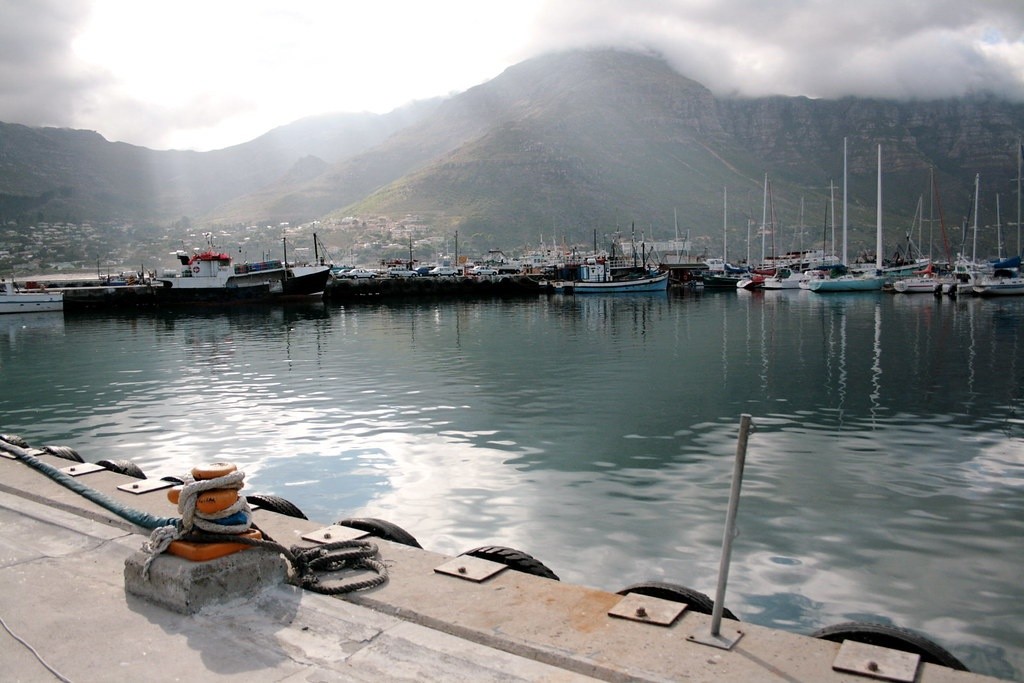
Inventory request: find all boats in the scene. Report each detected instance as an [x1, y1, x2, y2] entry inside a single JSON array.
[[52, 137, 1024, 315], [0, 273, 63, 314]]
